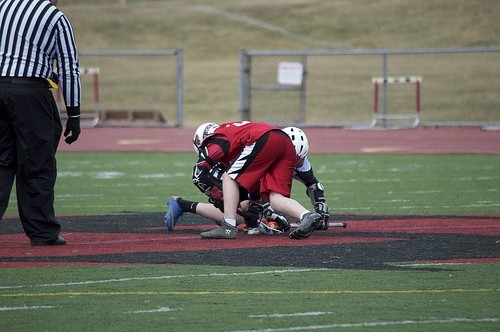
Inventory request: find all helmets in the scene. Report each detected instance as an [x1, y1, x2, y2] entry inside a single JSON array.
[[282, 126, 310, 160], [193, 122, 220, 160]]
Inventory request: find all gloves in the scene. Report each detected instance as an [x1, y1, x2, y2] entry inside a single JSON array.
[[213, 191, 224, 214], [244, 206, 262, 228], [265, 213, 289, 231], [312, 202, 330, 231], [64, 116, 82, 145]]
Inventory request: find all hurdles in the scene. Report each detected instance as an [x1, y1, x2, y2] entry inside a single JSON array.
[[55, 66, 100, 128], [342, 75, 423, 131]]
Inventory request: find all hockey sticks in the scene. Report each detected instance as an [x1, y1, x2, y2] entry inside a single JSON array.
[[236, 221, 347, 231]]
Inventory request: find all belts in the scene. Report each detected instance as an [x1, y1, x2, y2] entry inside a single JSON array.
[[0, 76, 48, 84]]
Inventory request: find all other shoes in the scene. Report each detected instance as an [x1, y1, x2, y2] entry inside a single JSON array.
[[30, 235, 68, 246]]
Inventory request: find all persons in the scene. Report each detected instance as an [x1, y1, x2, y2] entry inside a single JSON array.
[[164, 120, 330, 239], [0, 0, 82, 247]]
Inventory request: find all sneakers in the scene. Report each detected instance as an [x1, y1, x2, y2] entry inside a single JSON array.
[[288, 213, 323, 240], [164, 195, 184, 232], [200, 218, 239, 239]]
